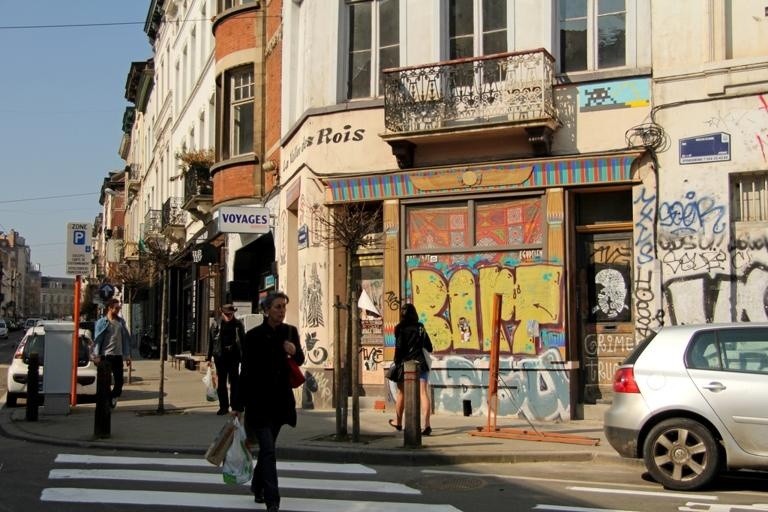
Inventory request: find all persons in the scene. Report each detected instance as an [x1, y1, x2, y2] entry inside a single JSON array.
[[205, 303, 246, 416], [92, 297, 132, 409], [230, 290, 305, 510], [386, 300, 433, 437]]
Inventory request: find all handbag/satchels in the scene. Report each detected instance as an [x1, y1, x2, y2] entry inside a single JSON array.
[[88, 339, 105, 367], [285, 324, 306, 388], [205, 418, 237, 467], [385, 366, 399, 381], [222, 417, 254, 485]]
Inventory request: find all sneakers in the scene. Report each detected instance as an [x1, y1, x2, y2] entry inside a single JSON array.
[[110, 396, 118, 409]]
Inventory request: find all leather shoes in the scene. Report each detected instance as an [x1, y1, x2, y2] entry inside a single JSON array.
[[389, 420, 402, 431], [216, 408, 229, 415], [254, 488, 266, 503], [267, 502, 280, 512], [421, 426, 432, 435]]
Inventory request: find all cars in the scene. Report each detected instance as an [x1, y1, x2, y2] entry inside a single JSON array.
[[604, 322, 767, 491], [0, 315, 98, 408]]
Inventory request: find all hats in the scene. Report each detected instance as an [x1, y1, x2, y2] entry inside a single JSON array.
[[218, 304, 238, 314]]
